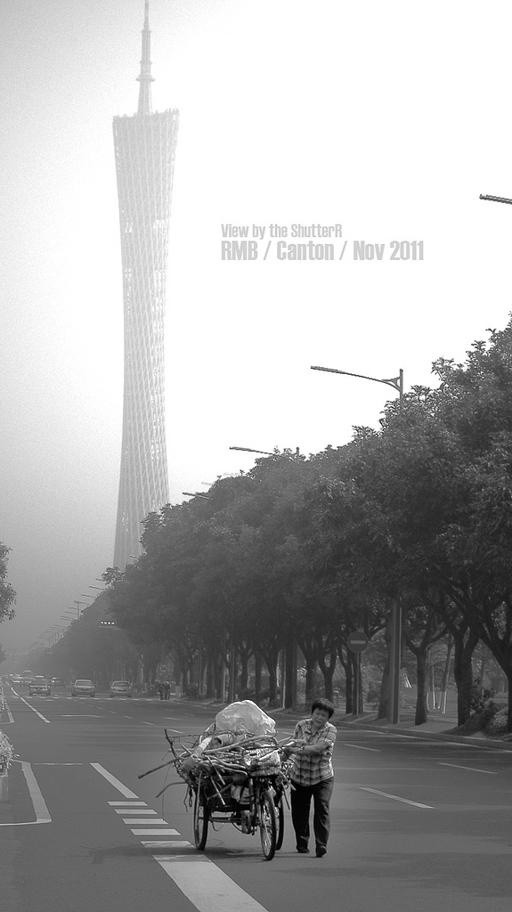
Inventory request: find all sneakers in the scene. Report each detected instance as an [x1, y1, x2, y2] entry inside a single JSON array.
[[296, 845, 328, 857]]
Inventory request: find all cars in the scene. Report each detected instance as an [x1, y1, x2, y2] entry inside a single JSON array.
[[71, 680, 96, 697], [110, 680, 132, 698], [10, 670, 62, 696]]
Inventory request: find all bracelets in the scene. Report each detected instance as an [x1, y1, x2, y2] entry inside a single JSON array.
[[299, 746, 304, 755]]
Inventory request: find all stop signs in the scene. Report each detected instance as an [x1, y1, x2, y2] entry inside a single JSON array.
[[347, 632, 369, 652]]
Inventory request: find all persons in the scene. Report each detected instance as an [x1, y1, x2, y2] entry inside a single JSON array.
[[157, 679, 172, 700], [280, 699, 338, 861]]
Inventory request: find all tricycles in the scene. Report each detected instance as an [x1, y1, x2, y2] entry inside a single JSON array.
[[192, 744, 296, 860]]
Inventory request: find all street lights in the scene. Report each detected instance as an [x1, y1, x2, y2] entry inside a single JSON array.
[[311, 364, 404, 728], [229, 445, 363, 715]]
